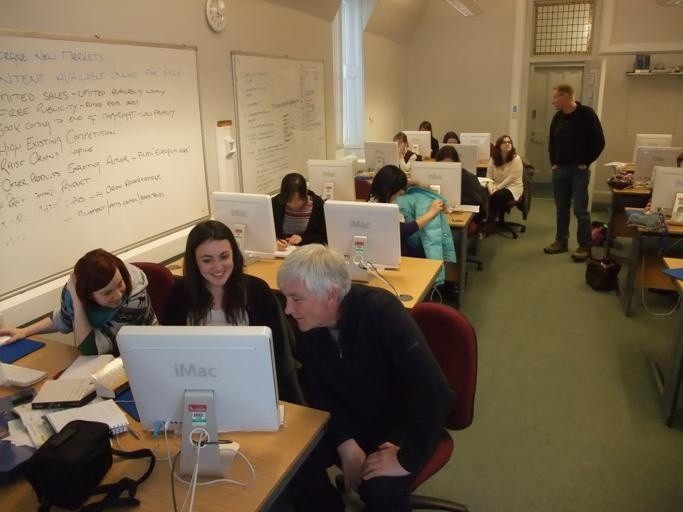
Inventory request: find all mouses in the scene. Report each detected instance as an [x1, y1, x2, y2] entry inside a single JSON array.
[[0, 335, 12, 347]]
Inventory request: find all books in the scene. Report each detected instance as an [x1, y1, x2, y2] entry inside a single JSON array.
[[659, 267, 683, 281], [0, 352, 139, 451]]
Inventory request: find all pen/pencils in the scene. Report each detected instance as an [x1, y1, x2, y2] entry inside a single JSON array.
[[52, 356, 74, 379], [126, 424, 142, 441]]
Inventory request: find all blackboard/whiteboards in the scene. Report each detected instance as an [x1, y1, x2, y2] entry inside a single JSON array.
[[0, 29, 212, 303], [232, 50, 327, 196]]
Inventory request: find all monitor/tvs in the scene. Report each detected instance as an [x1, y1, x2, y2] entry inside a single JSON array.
[[323, 200, 400, 282], [402, 130, 431, 159], [411, 161, 462, 213], [363, 141, 399, 171], [116, 326, 280, 477], [634, 146, 683, 178], [650, 167, 683, 225], [460, 133, 490, 162], [439, 143, 477, 176], [212, 191, 275, 265], [307, 160, 356, 202], [634, 134, 672, 163]]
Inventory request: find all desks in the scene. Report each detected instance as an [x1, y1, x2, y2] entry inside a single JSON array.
[[602, 160, 683, 427]]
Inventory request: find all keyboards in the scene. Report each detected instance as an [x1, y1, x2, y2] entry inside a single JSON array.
[[245, 243, 297, 259], [1, 363, 48, 389], [368, 264, 400, 270]]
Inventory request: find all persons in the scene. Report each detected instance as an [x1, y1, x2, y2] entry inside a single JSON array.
[[643, 152, 682, 213], [393, 121, 523, 260], [362, 166, 443, 256], [543, 82, 605, 260], [277, 239, 453, 512], [1, 248, 161, 359], [166, 221, 301, 405], [269, 172, 326, 250]]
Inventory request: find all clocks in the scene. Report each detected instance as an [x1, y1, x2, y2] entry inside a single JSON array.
[[204, 0, 228, 33]]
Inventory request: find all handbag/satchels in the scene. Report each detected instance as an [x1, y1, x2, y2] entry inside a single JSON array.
[[585, 262, 618, 291], [24, 420, 155, 512]]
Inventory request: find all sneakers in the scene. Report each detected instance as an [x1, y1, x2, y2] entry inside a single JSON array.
[[544, 241, 568, 253], [571, 247, 591, 259]]
